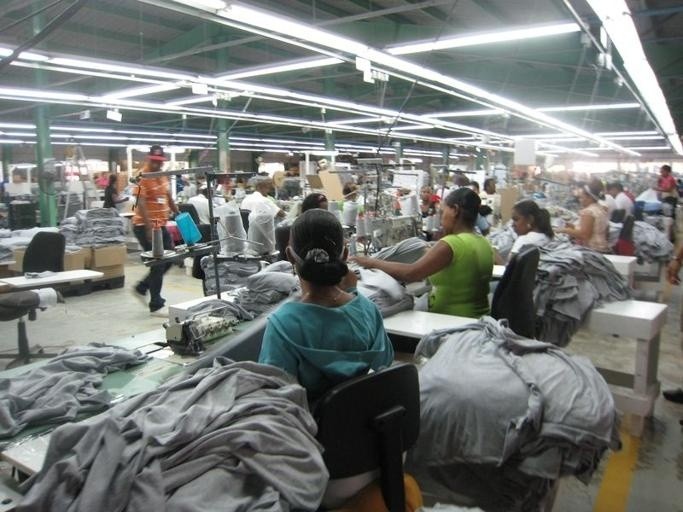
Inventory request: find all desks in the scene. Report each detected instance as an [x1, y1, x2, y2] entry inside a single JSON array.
[[0, 215, 683, 512]]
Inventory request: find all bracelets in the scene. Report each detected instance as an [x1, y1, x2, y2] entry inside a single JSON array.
[[671, 256, 680, 260]]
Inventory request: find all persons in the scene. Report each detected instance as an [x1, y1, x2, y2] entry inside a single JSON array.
[[131, 145, 183, 310], [397, 174, 502, 237], [512, 172, 552, 200], [664, 225, 682, 285], [175, 172, 218, 225], [347, 188, 495, 317], [103, 174, 130, 209], [654, 165, 679, 241], [557, 181, 636, 254], [301, 193, 328, 213], [242, 176, 289, 253], [256, 206, 407, 511], [343, 182, 364, 226], [506, 202, 554, 263]]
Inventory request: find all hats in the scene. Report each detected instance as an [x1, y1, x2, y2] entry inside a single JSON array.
[[145, 145, 170, 161]]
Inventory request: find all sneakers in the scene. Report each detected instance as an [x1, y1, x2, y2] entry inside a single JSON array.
[[132, 285, 149, 307], [149, 305, 169, 317]]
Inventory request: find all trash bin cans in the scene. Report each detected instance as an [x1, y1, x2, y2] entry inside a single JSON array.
[[7, 200, 36, 230], [174, 212, 201, 244]]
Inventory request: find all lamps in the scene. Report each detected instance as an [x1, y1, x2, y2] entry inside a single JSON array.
[[0, 0, 683, 158]]
